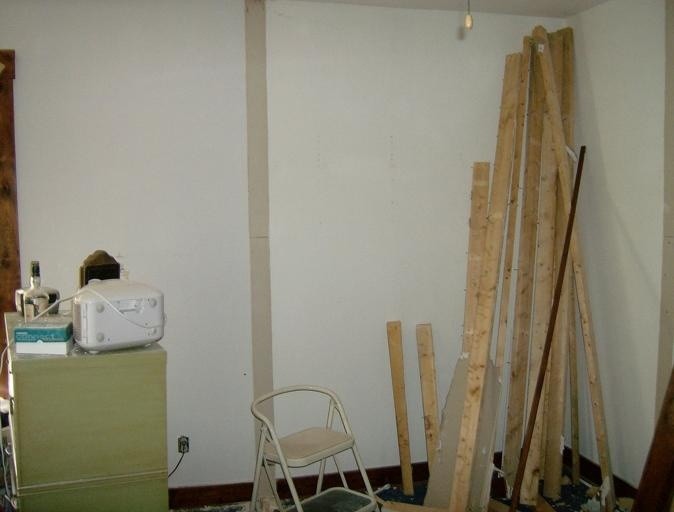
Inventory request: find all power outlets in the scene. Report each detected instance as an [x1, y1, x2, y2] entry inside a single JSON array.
[[177, 436, 189, 452]]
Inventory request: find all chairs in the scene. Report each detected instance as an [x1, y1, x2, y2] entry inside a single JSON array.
[[249, 384, 380, 512]]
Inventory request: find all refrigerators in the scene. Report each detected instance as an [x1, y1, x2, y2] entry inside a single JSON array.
[[3, 309, 168, 511]]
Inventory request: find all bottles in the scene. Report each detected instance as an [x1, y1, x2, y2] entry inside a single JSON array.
[[23, 261, 49, 323]]
